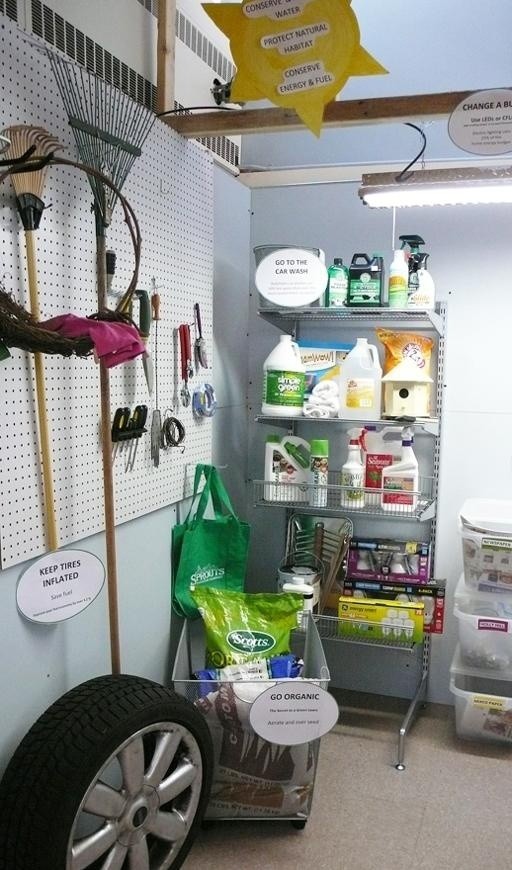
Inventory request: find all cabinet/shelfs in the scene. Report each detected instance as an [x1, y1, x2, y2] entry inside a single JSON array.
[[250, 298, 448, 771]]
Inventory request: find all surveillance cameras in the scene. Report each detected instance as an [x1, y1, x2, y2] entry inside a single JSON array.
[[211, 75, 245, 105]]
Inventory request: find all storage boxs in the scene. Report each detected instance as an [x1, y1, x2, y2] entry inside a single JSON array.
[[454, 573, 512, 671], [460, 499, 512, 593], [449, 643, 512, 747]]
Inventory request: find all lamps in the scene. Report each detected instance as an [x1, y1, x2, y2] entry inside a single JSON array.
[[357, 167, 511, 208]]
